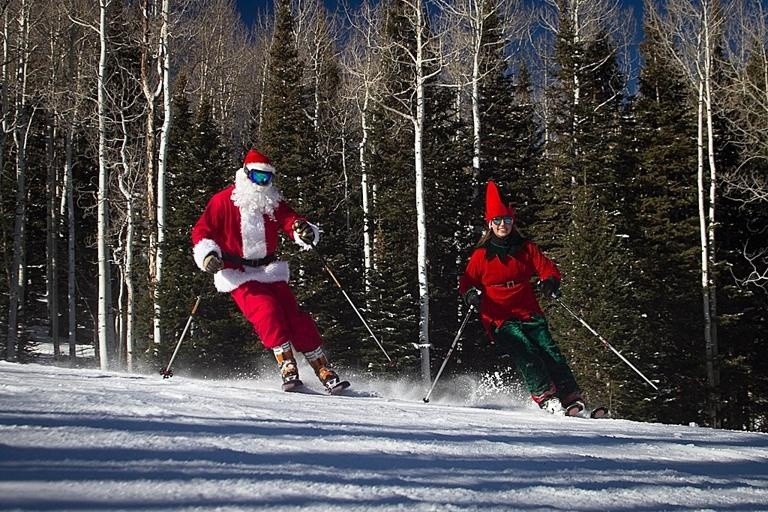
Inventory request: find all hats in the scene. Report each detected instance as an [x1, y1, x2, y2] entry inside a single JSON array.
[[242, 147, 277, 172], [484, 179, 516, 224]]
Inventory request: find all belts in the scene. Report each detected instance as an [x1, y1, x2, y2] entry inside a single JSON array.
[[493, 279, 522, 288]]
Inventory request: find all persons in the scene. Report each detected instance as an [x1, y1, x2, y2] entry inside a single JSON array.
[[193, 148, 341, 390], [459, 179, 589, 416]]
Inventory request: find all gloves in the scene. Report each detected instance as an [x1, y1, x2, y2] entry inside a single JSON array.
[[539, 276, 561, 297], [204, 256, 224, 274], [291, 220, 315, 243], [465, 288, 480, 311]]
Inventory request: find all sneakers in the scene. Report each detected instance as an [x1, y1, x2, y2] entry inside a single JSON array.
[[538, 395, 564, 414], [571, 398, 587, 414]]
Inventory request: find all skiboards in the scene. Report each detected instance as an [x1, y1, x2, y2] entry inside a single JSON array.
[[281, 379, 351, 395], [565, 403, 608, 420]]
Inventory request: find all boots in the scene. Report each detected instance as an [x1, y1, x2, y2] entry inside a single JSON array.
[[303, 347, 339, 386], [272, 342, 300, 381]]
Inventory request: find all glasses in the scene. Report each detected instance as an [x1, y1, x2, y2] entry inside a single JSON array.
[[249, 169, 274, 185], [493, 216, 514, 226]]
